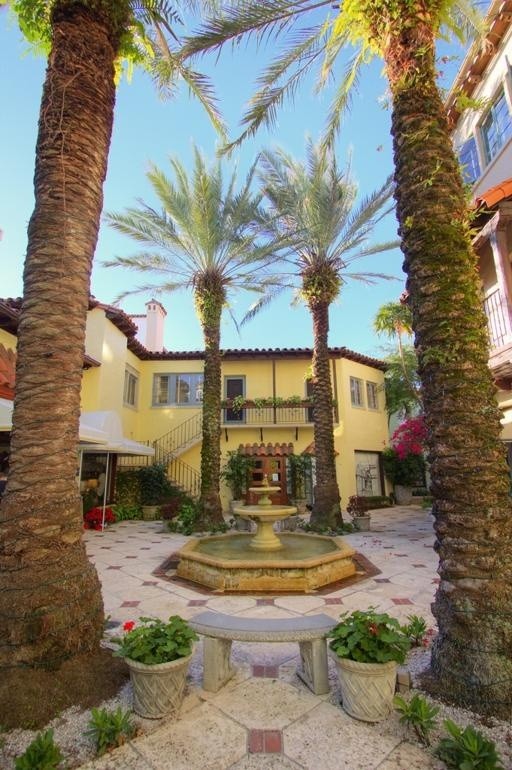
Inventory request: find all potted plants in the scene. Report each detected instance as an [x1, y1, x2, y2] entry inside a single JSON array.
[[279, 449, 315, 515], [134, 462, 170, 521], [381, 447, 427, 507], [219, 449, 257, 515]]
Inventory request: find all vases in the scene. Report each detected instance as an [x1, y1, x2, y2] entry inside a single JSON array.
[[333, 656, 400, 722], [162, 518, 181, 531], [351, 515, 373, 530], [124, 648, 193, 722]]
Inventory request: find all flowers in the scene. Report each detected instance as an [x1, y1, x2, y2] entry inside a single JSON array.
[[106, 615, 203, 666], [347, 495, 371, 515], [157, 498, 182, 518], [325, 601, 440, 664]]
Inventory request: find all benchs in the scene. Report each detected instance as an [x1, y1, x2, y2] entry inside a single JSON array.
[[186, 610, 340, 697]]
